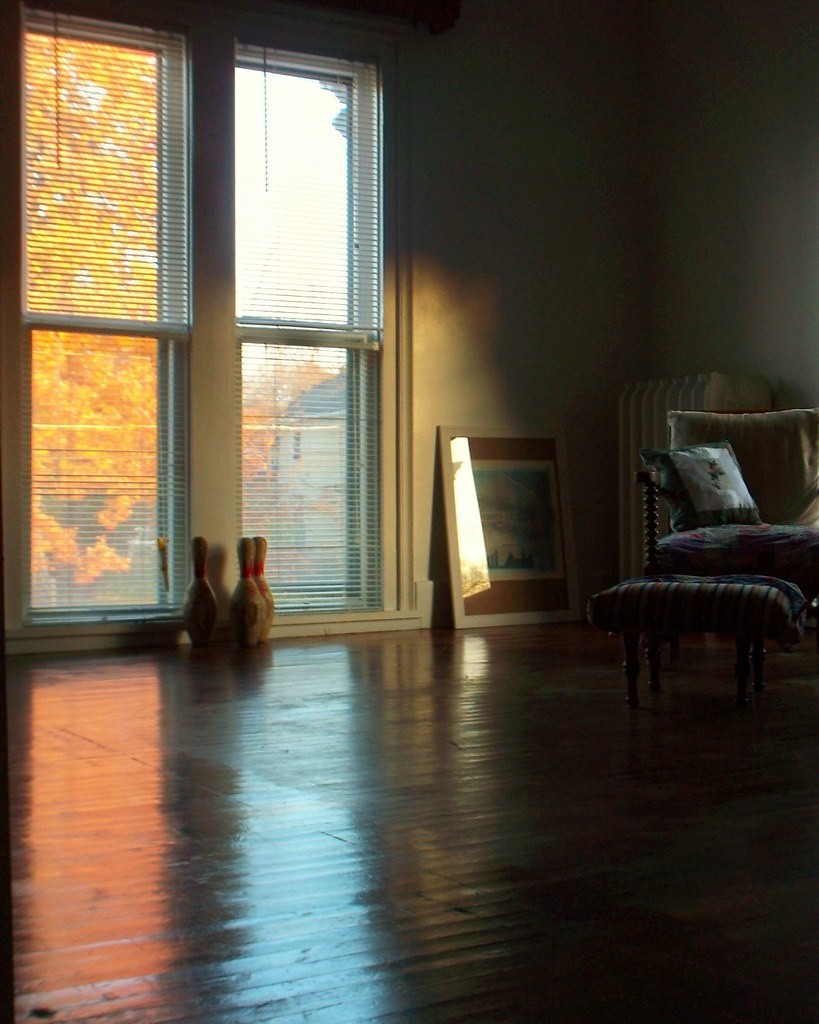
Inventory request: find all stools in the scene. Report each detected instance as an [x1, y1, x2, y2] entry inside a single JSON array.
[[587, 573, 807, 707]]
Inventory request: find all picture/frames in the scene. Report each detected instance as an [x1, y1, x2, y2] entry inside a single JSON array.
[[436, 425, 582, 629]]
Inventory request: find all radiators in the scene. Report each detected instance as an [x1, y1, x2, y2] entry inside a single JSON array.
[[618, 371, 739, 587]]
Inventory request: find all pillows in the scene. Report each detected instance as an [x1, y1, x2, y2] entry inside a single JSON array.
[[639, 438, 764, 535]]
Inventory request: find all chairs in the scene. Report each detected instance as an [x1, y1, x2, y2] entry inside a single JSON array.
[[635, 407, 819, 695]]
